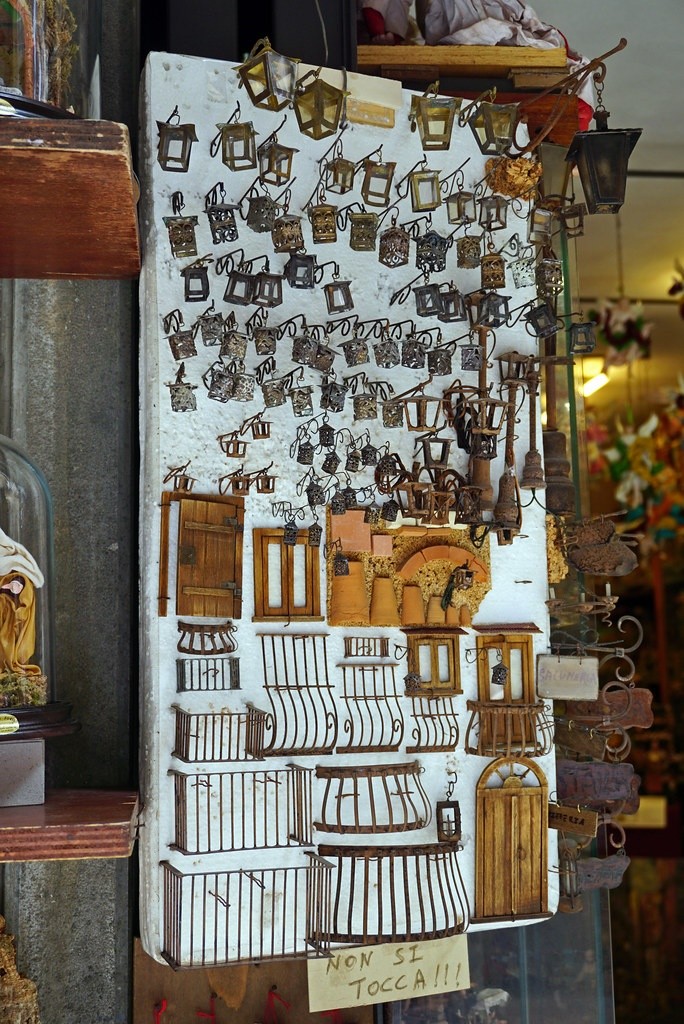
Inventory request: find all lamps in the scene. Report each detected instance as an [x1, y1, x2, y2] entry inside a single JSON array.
[[505, 38, 644, 216]]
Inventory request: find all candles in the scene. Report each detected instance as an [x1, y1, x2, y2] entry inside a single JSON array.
[[606, 581, 611, 598], [550, 588, 555, 600], [580, 592, 585, 602]]
